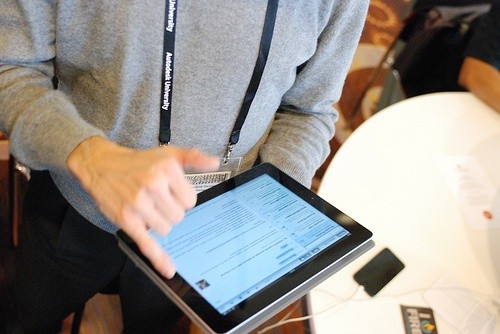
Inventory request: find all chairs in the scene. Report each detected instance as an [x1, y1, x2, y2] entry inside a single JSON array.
[[371, 71, 407, 110], [454, 58, 500, 112]]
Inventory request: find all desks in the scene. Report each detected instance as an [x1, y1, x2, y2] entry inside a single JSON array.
[[304, 91, 499, 334]]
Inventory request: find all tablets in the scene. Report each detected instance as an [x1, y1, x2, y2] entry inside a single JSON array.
[[116, 162, 373, 334]]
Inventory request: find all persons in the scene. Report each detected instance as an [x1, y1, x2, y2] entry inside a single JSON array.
[[0, 0, 370, 334]]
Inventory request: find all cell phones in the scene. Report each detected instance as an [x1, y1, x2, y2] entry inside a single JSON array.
[[354, 248, 405, 297]]
